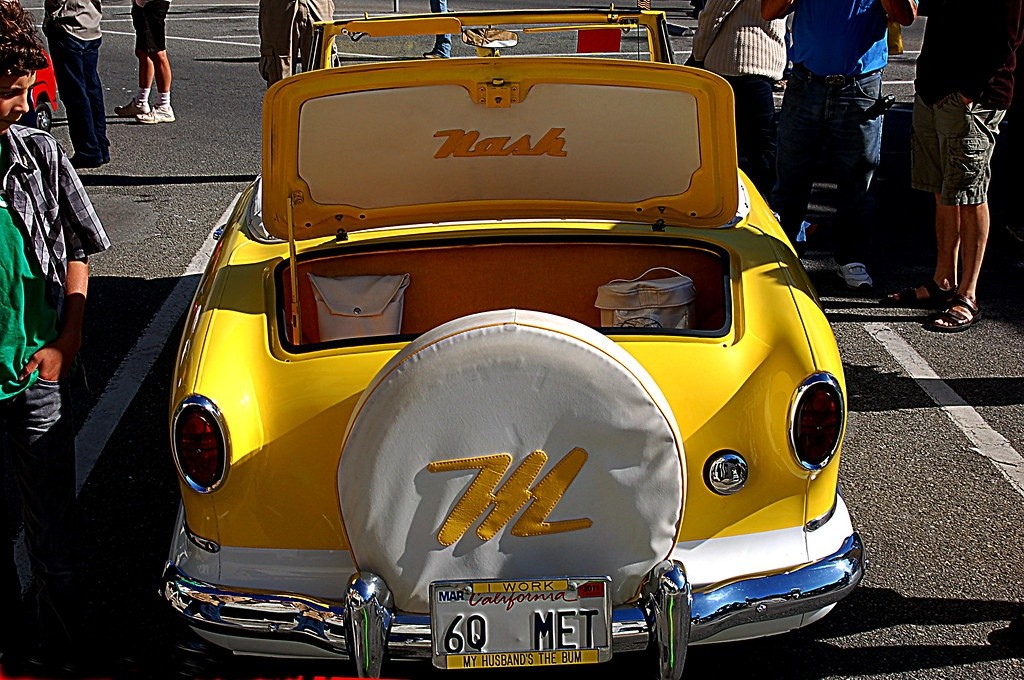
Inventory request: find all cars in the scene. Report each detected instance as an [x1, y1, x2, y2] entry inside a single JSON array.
[[156, 4, 865, 680], [18, 45, 60, 136]]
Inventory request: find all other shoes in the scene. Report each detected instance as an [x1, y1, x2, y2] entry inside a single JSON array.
[[69, 157, 103, 168], [104, 158, 110, 163]]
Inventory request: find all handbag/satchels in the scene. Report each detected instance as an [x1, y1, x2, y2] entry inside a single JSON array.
[[682, 51, 709, 69]]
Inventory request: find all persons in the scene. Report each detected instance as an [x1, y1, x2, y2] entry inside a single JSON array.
[[424, 0, 452, 59], [114, 0, 176, 123], [43, 0, 110, 169], [0, 0, 112, 664], [258, 0, 342, 89], [691, 0, 1024, 332]]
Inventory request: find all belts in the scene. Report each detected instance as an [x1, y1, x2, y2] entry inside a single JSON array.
[[797, 64, 878, 87]]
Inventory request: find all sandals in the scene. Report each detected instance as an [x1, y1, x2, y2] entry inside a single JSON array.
[[934, 294, 984, 332], [887, 279, 960, 306]]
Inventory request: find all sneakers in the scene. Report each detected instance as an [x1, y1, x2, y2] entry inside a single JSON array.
[[113, 98, 152, 117], [837, 263, 873, 292], [134, 103, 176, 124]]
